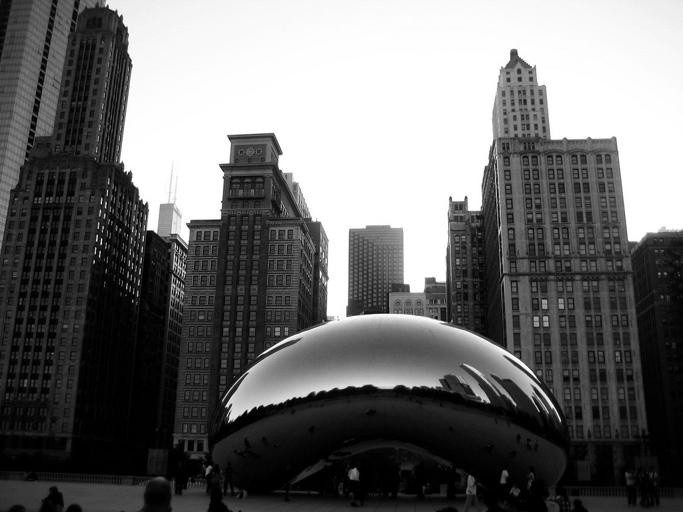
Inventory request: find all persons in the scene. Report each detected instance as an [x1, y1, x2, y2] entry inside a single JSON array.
[[623, 465, 662, 508], [347, 464, 364, 507], [7, 462, 236, 511], [462, 466, 588, 512]]
